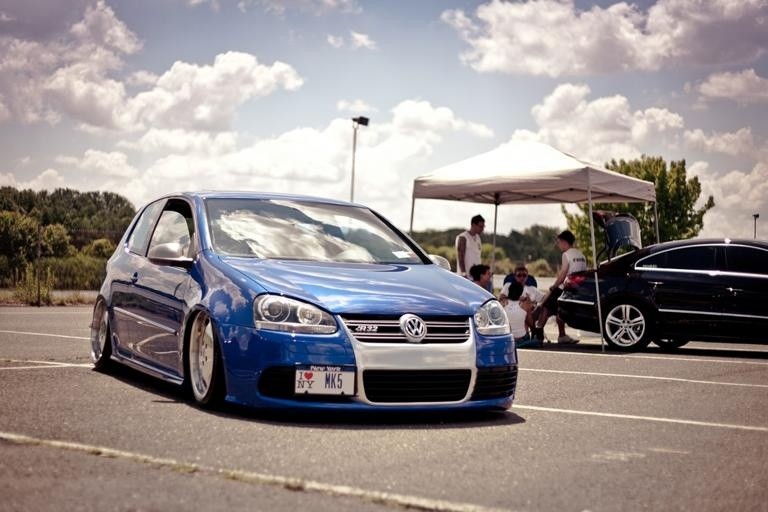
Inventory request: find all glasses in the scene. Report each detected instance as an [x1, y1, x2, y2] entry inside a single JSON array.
[[515, 273, 527, 278]]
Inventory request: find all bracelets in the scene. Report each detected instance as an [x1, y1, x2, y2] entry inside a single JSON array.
[[461, 272, 468, 276]]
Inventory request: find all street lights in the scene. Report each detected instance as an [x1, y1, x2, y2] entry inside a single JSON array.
[[752, 213, 760, 239], [350, 116, 369, 203]]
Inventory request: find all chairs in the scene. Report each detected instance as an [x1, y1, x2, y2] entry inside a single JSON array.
[[503, 273, 537, 289]]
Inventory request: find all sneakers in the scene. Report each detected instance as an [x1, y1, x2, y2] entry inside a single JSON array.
[[557, 334, 580, 344]]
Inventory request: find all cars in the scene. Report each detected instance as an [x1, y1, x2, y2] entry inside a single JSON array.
[[556, 235, 768, 354], [88, 189, 519, 417]]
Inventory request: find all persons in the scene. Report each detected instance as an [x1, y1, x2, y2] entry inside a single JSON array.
[[524, 230, 586, 347], [500, 282, 534, 347], [470, 264, 494, 294], [500, 267, 580, 344], [455, 214, 485, 282]]
